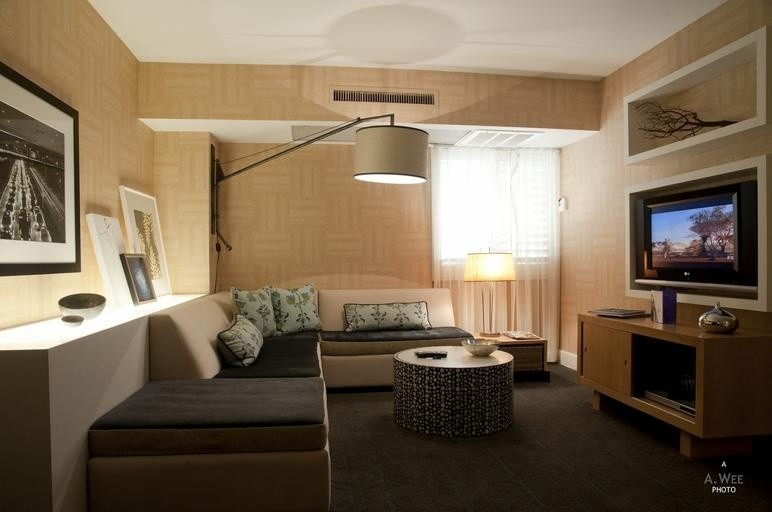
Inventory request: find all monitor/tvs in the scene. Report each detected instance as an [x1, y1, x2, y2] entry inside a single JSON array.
[[635, 179, 758, 288]]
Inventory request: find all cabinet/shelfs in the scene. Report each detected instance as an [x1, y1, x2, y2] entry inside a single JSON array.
[[622, 24, 767, 166], [623, 152, 770, 315], [573, 306, 772, 461], [479, 329, 551, 383]]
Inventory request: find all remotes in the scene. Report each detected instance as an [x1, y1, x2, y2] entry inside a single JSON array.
[[416, 352, 447, 358]]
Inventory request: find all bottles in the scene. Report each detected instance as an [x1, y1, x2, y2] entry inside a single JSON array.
[[697, 301, 739, 333]]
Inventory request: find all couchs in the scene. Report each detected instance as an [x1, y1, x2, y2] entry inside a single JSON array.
[[318, 285, 473, 391], [83, 287, 334, 509]]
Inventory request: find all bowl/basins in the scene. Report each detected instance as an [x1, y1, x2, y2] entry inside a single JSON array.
[[461, 338, 508, 356], [59, 294, 106, 319], [62, 315, 82, 327]]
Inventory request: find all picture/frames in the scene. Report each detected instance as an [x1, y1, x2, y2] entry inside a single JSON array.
[[119, 182, 172, 297], [0, 57, 82, 276], [119, 252, 157, 307]]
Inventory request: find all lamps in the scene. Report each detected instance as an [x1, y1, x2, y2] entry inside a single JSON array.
[[210, 112, 429, 250], [459, 247, 529, 338]]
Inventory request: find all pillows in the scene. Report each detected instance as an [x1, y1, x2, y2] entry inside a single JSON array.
[[217, 312, 265, 369], [268, 279, 321, 336], [231, 283, 281, 339], [343, 301, 433, 333]]
